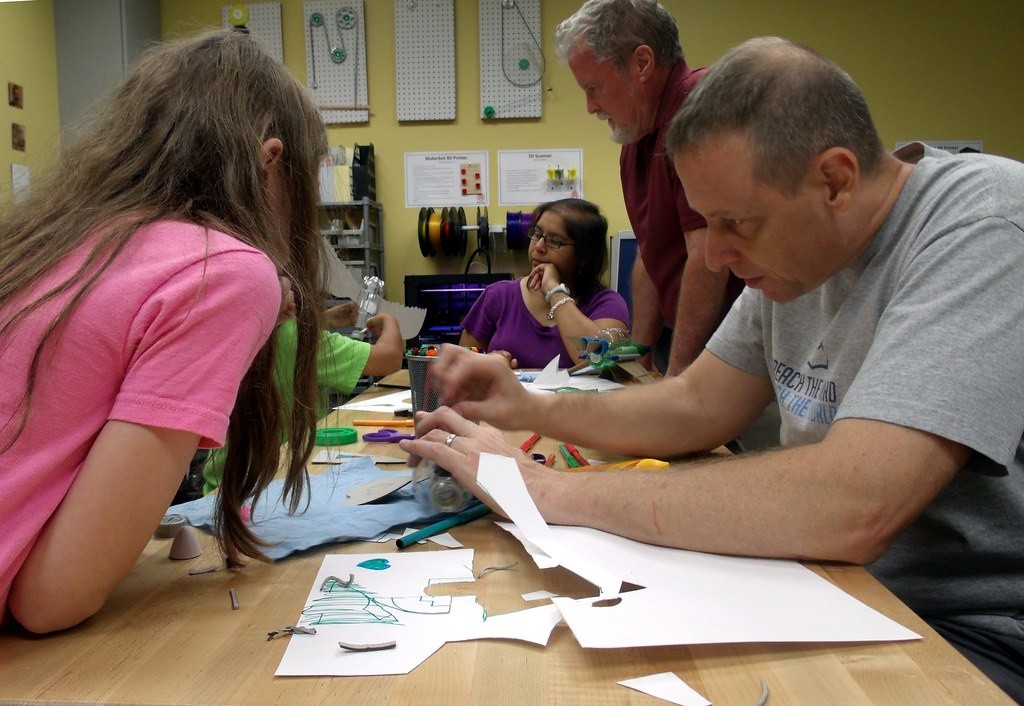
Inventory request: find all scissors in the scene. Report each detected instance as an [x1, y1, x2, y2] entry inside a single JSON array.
[[362, 428, 419, 445]]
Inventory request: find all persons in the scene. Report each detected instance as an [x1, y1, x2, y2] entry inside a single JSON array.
[[391, 31, 1023, 704], [0, 22, 347, 660], [195, 289, 417, 505], [538, 0, 761, 420], [451, 191, 634, 376]]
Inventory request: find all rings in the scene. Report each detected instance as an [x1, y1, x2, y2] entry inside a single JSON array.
[[444, 433, 457, 447]]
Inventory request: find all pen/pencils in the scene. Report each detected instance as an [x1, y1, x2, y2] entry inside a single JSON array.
[[564, 440, 592, 466], [518, 433, 546, 453], [395, 503, 497, 549], [404, 344, 479, 416], [352, 419, 416, 428], [558, 443, 580, 469]]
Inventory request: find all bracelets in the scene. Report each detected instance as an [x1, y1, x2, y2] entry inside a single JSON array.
[[547, 297, 576, 323]]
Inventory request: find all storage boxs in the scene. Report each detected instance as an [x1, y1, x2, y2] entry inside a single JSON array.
[[407, 344, 488, 432]]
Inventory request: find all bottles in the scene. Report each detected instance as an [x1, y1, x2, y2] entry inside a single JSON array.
[[349, 276, 385, 340], [412, 458, 473, 514]]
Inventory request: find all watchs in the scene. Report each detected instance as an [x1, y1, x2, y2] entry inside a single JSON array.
[[545, 283, 572, 305]]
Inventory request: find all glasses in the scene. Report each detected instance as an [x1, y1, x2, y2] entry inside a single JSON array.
[[527, 227, 575, 250]]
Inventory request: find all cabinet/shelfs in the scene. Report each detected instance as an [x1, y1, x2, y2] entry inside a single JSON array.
[[311, 198, 384, 411]]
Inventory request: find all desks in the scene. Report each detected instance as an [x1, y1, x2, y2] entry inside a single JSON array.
[[1, 368, 1019, 705]]
